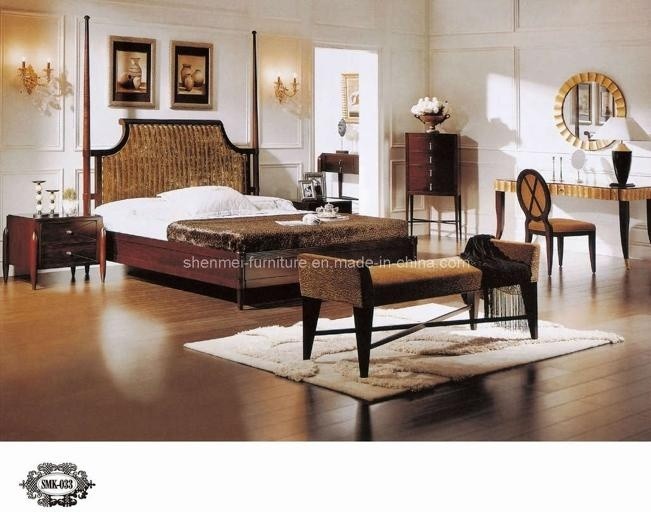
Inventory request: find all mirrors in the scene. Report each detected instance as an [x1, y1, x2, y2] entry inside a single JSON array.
[[554, 71, 627, 154]]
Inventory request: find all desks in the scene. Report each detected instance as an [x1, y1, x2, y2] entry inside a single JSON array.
[[318, 152, 360, 201], [494, 177, 651, 268], [403, 131, 461, 242]]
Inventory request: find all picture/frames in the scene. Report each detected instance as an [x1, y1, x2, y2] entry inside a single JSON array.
[[573, 82, 592, 124], [170, 40, 214, 110], [342, 72, 359, 125], [304, 172, 326, 198], [299, 180, 316, 200], [598, 84, 617, 125], [109, 35, 157, 109]]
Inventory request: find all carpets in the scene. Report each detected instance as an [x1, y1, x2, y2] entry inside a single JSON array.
[[184, 303, 623, 404]]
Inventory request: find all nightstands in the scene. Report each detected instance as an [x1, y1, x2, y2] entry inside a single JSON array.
[[291, 200, 354, 215], [3, 214, 107, 289]]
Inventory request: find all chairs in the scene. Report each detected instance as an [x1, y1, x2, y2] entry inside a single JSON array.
[[516, 169, 597, 276]]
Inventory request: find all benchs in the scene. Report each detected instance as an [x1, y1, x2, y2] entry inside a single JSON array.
[[300, 236, 540, 378]]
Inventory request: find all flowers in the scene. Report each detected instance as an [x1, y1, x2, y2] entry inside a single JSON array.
[[411, 95, 453, 114]]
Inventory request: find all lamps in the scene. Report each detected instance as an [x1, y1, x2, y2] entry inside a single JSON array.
[[274, 66, 287, 108], [17, 59, 37, 97], [589, 118, 651, 187], [286, 72, 298, 98], [42, 59, 52, 84]]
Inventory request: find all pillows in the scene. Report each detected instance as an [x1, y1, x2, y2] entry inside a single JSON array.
[[156, 185, 260, 217]]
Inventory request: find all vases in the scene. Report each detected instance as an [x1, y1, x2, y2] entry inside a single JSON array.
[[418, 115, 450, 131]]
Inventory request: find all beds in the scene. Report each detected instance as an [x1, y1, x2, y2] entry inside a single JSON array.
[[82, 16, 418, 311]]
